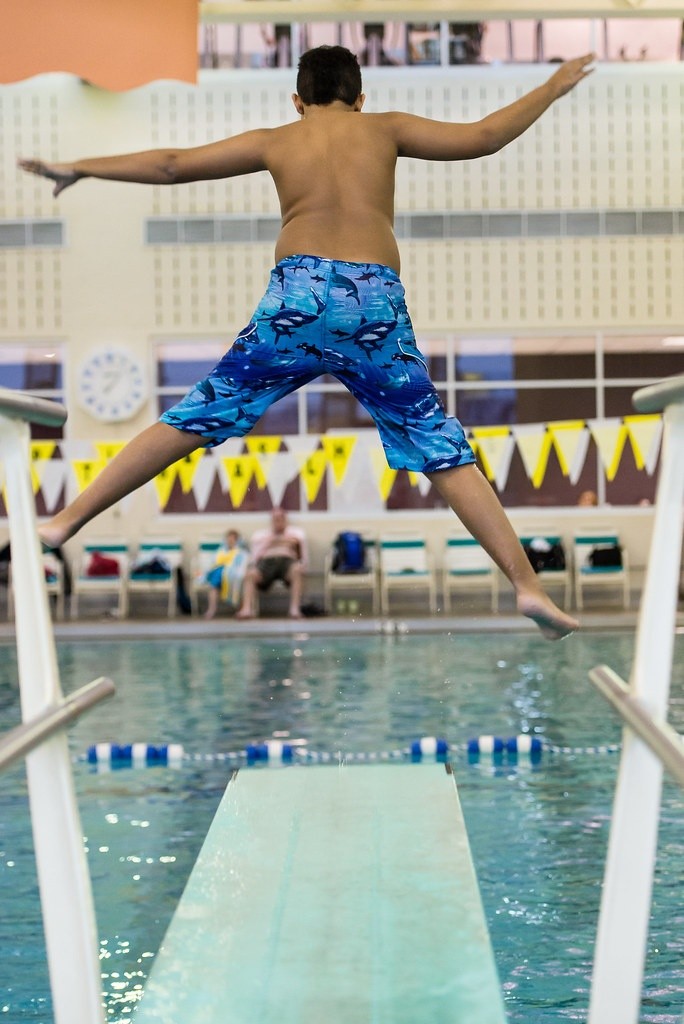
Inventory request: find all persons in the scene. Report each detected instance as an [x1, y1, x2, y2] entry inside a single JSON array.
[[17, 42, 599, 643], [199, 506, 308, 621]]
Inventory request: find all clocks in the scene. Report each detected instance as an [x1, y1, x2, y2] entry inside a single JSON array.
[[75, 347, 148, 421]]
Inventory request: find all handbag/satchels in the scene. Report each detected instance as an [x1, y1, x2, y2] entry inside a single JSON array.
[[589, 545, 623, 569], [528, 544, 565, 575]]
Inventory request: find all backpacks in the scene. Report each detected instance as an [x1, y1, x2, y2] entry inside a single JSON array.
[[332, 532, 368, 574]]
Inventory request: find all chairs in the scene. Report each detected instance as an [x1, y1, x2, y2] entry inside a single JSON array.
[[518, 534, 573, 609], [574, 531, 630, 611], [125, 537, 183, 618], [190, 539, 244, 618], [380, 530, 438, 614], [325, 533, 380, 615], [7, 541, 65, 621], [72, 540, 129, 618], [444, 533, 499, 615]]
